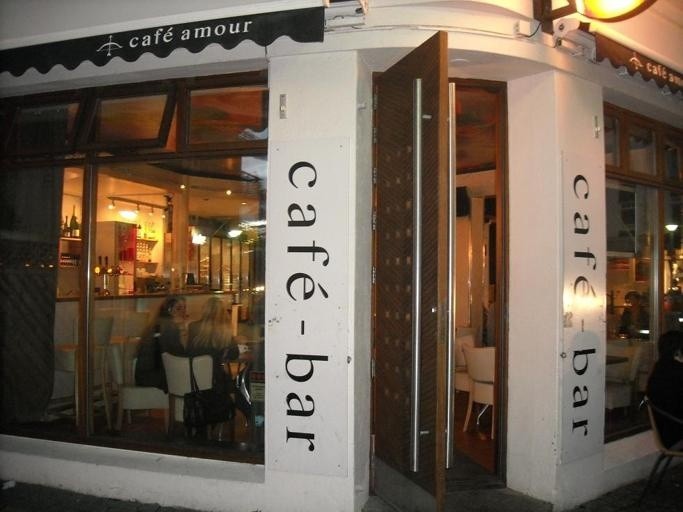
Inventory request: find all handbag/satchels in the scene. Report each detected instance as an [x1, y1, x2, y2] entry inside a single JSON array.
[[182, 386, 237, 428]]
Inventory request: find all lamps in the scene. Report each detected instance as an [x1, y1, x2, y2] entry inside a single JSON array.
[[530, 0, 659, 36]]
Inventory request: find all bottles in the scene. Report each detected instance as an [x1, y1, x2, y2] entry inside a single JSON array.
[[63, 204, 81, 239]]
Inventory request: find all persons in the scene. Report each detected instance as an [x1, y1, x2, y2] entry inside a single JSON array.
[[134, 295, 189, 393], [187, 296, 257, 427], [647, 329, 683, 453], [619, 291, 649, 340]]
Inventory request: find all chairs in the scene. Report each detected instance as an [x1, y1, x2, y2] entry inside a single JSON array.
[[452, 336, 496, 442], [54, 306, 214, 442], [605, 341, 681, 499]]
